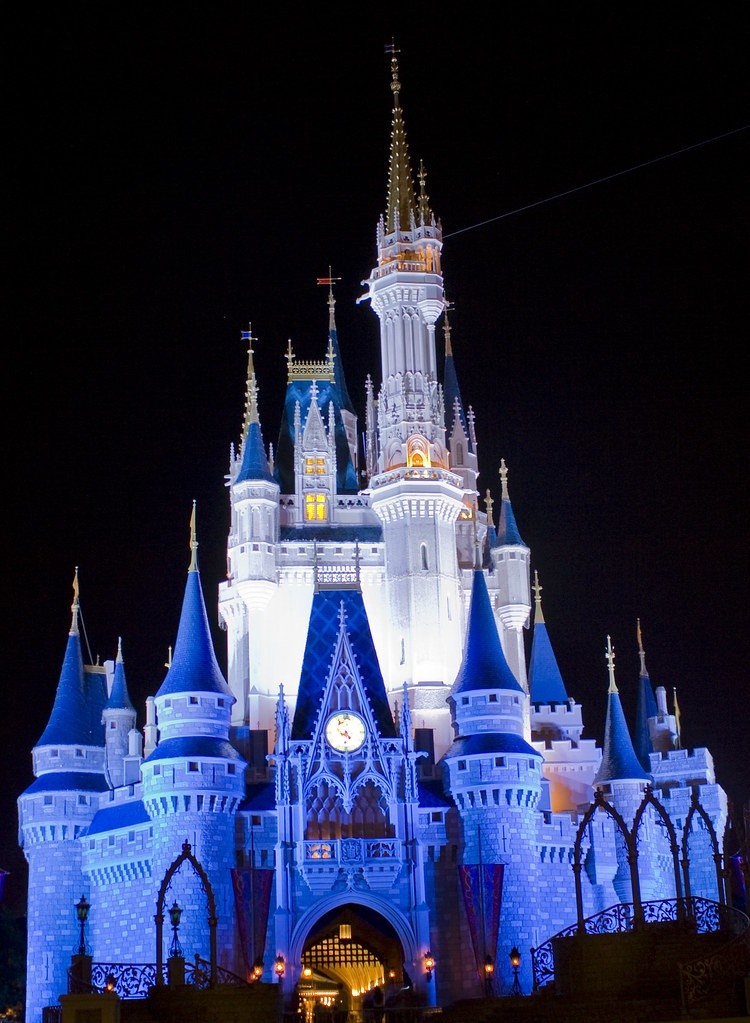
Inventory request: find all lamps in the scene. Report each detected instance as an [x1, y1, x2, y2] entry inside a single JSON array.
[[339, 917, 352, 949], [247, 959, 264, 985], [423, 949, 437, 980], [508, 947, 523, 999], [275, 954, 287, 982], [483, 953, 498, 997]]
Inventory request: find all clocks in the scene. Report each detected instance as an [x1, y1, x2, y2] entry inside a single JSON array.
[[324, 709, 369, 755]]
